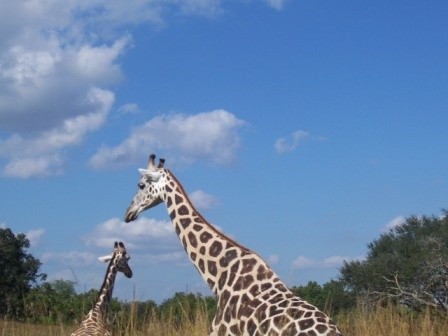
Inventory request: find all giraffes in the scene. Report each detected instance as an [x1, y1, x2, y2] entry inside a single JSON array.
[[124, 154, 344, 336], [70, 241, 132, 336]]
